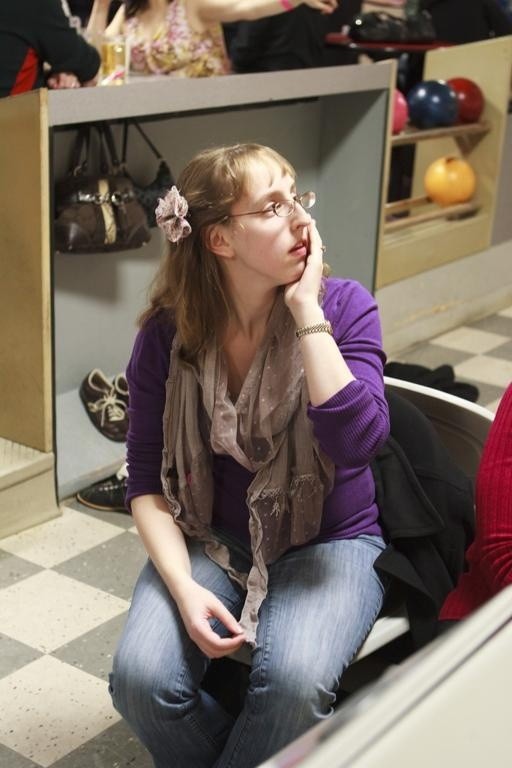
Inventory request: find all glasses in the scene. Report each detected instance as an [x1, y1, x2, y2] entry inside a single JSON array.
[[227, 191, 317, 220]]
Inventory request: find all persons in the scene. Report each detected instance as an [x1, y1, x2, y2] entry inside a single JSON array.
[[106, 144, 394, 766], [1, 0, 101, 99], [433, 377, 512, 634], [83, 1, 337, 88]]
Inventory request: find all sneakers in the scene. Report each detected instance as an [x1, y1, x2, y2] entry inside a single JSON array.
[[79, 369, 132, 441], [79, 476, 129, 512]]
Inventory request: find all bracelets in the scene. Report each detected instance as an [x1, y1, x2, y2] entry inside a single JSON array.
[[281, 1, 293, 12], [296, 320, 334, 340]]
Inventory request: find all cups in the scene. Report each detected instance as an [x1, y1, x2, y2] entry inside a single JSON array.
[[99, 33, 126, 87]]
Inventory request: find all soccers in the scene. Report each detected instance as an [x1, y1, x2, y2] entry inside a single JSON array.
[[425, 157, 475, 205], [391, 78, 487, 134]]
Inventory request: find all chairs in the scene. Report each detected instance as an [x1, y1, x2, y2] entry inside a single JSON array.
[[224, 373, 499, 670]]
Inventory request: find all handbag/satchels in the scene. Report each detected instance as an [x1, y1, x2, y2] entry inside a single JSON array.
[[54, 122, 177, 255], [348, 8, 437, 44]]
